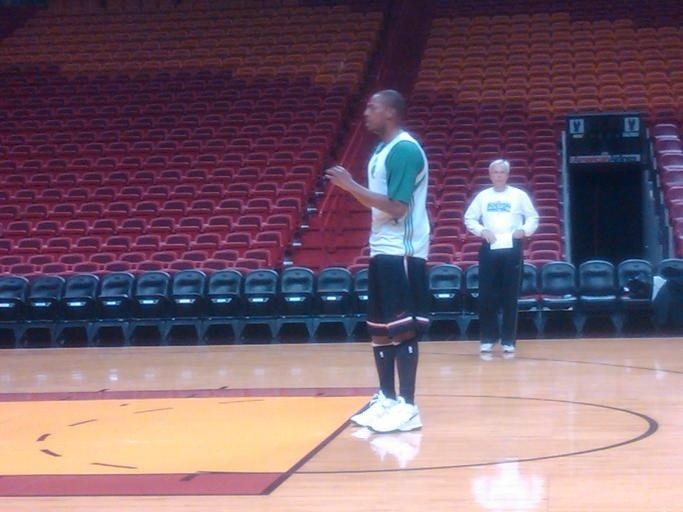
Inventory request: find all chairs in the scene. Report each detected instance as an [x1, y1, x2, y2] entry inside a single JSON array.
[[404, 2, 683, 339], [0, 0, 388, 345]]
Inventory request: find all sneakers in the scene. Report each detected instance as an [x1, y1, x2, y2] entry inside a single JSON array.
[[349, 388, 402, 427], [365, 394, 423, 433], [479, 343, 494, 353], [502, 344, 516, 352]]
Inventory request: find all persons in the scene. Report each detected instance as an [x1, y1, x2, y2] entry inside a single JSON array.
[[325, 89, 431, 433], [465, 159, 539, 351]]
